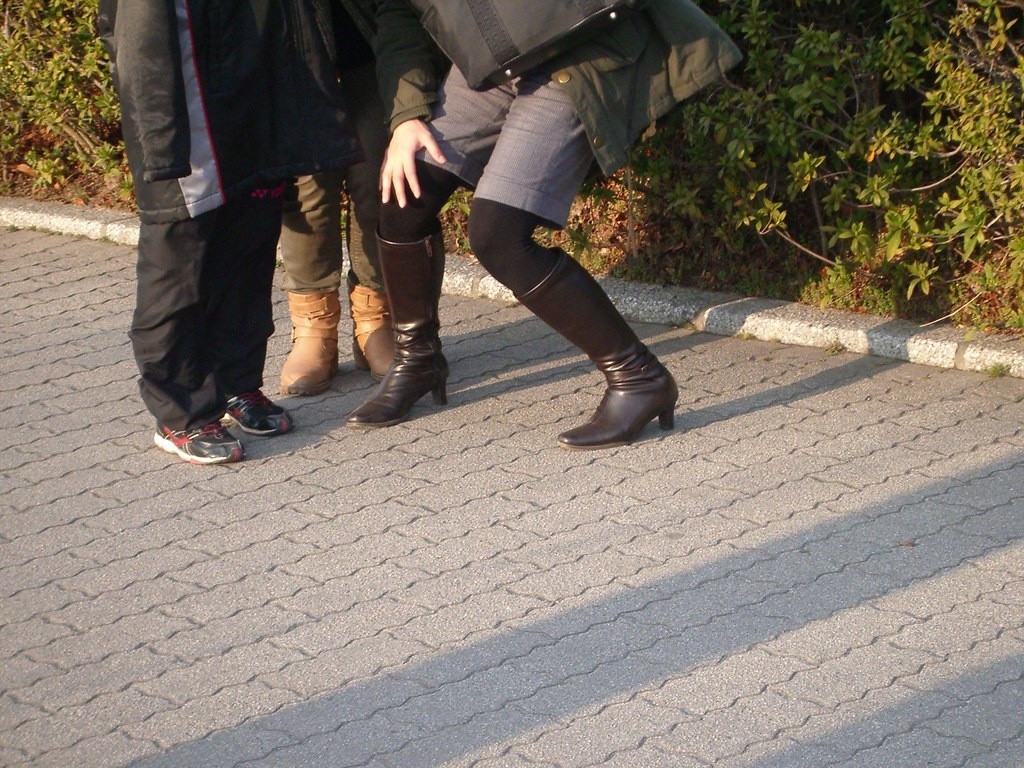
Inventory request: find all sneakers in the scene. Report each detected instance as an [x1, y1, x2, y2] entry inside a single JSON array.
[[222, 389, 294, 436], [153, 415, 245, 465]]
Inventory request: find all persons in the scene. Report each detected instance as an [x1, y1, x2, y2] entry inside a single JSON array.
[[97, 0, 397, 465], [345, 0, 743, 452]]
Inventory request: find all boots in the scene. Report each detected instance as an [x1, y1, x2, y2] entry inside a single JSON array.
[[345, 214, 449, 427], [347, 269, 396, 383], [279, 291, 341, 395], [513, 246, 679, 450]]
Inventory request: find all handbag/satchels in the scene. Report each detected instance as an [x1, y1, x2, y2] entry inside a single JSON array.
[[410, 0, 619, 92]]
